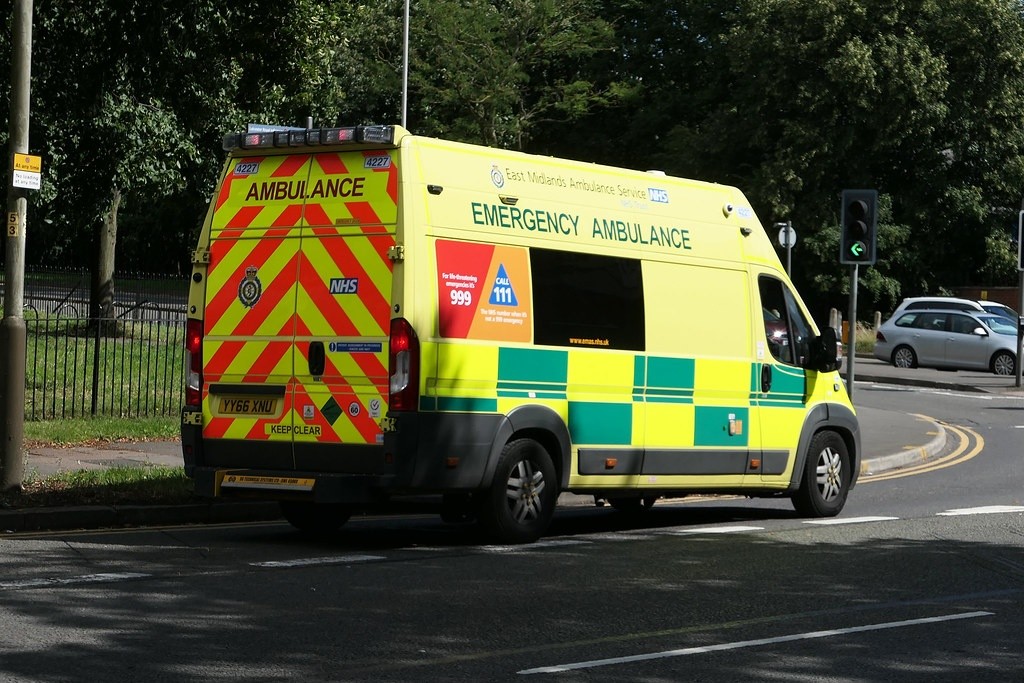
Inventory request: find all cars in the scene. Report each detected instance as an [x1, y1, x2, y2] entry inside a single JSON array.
[[763, 307, 790, 346], [873, 309, 1023, 375]]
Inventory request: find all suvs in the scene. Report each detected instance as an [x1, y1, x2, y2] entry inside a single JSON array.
[[892, 297, 1020, 324]]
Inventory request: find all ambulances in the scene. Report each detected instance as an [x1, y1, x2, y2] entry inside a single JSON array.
[[179, 116, 863, 544]]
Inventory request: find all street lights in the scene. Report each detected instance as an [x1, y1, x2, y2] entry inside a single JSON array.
[[774, 222, 792, 281]]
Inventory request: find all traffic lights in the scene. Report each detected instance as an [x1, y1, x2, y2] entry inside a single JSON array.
[[840, 189, 876, 265]]
